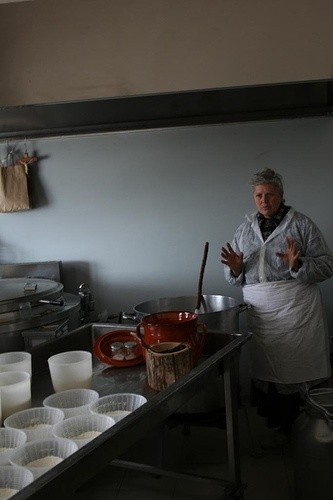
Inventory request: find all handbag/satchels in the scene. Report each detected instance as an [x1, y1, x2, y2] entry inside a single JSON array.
[[0, 153, 34, 211]]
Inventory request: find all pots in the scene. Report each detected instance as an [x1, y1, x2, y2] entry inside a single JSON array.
[[0, 276, 63, 312], [122, 295, 253, 415]]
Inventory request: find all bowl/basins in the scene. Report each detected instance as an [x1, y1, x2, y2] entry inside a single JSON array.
[[0, 465, 35, 500], [51, 414, 116, 448], [8, 437, 79, 478], [0, 427, 28, 466], [43, 389, 99, 419], [3, 407, 64, 442], [87, 393, 149, 423]]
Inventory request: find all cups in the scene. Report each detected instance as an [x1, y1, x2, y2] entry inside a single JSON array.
[[144, 341, 193, 390], [1, 372, 32, 420], [47, 350, 94, 392], [0, 351, 32, 375]]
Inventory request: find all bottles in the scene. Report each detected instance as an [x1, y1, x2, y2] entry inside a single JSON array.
[[136, 309, 208, 368]]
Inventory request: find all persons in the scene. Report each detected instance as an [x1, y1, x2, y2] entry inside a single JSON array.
[[219, 166, 333, 437]]
